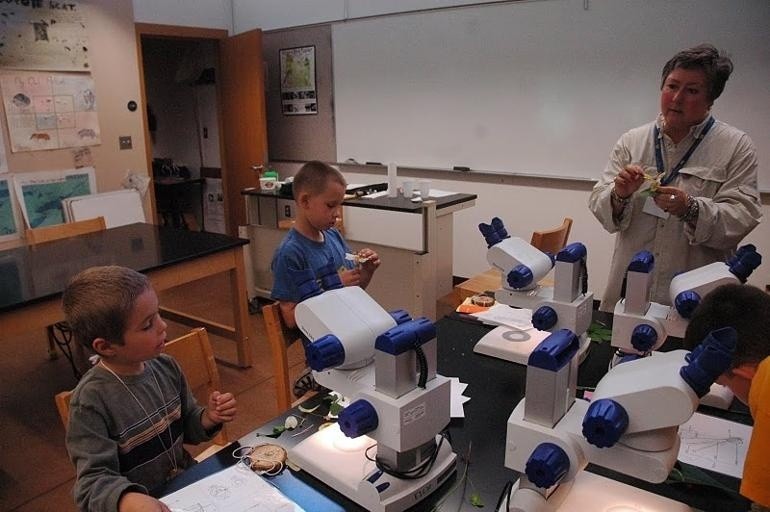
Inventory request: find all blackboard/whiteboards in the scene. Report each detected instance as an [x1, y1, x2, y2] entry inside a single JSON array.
[[331, 1, 770, 192]]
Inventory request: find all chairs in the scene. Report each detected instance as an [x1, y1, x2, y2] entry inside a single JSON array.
[[55, 324, 230, 462], [261, 298, 308, 413], [26, 217, 108, 244], [452, 217, 577, 310]]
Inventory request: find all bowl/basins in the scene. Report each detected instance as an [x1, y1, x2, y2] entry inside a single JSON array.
[[259, 178, 275, 190]]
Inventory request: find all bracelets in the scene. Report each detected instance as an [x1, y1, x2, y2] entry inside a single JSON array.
[[679, 195, 698, 224], [613, 188, 630, 207]]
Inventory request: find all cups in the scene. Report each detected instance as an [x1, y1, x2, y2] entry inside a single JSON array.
[[419, 180, 432, 197], [402, 179, 414, 198]]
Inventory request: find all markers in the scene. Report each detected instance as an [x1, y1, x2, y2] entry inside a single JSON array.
[[366, 163, 381, 165]]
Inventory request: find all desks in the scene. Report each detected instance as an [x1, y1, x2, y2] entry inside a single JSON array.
[[0, 223, 255, 373], [240, 177, 482, 332], [87, 287, 769, 512]]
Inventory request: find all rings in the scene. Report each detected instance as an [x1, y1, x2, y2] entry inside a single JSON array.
[[664, 208, 668, 213], [671, 194, 675, 201]]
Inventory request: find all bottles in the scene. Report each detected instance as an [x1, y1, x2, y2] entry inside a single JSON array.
[[388, 160, 397, 198]]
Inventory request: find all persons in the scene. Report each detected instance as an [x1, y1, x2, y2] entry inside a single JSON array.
[[588, 43, 762, 313], [271, 160, 381, 398], [685, 285, 769, 512], [63, 266, 237, 512]]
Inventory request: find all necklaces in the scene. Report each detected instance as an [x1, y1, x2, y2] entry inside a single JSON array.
[[102, 361, 182, 473]]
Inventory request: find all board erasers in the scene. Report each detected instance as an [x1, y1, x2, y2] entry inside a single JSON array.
[[454, 167, 469, 171]]
